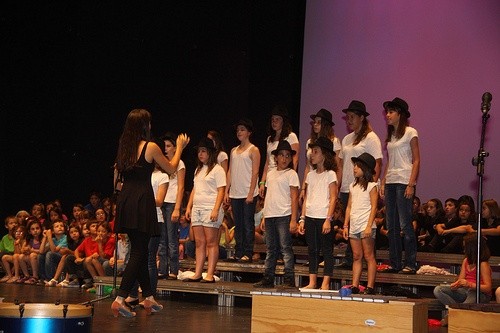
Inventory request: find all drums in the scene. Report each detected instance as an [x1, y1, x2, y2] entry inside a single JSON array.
[[1, 302, 93, 333]]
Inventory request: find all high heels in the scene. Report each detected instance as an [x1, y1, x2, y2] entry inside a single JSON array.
[[125, 299, 139, 309], [111, 301, 131, 318], [144, 300, 163, 313]]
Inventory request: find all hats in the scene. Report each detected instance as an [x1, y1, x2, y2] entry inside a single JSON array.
[[232, 118, 256, 135], [342, 100, 370, 117], [163, 135, 177, 147], [383, 97, 411, 118], [310, 108, 335, 126], [271, 140, 296, 155], [268, 106, 288, 116], [197, 139, 217, 151], [351, 152, 377, 174], [308, 136, 336, 155], [201, 121, 219, 132]]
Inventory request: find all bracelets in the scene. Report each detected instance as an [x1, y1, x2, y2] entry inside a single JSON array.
[[343, 225, 348, 228], [299, 215, 305, 219], [259, 182, 265, 185], [471, 282, 472, 288], [299, 220, 305, 223], [326, 216, 332, 220], [408, 184, 416, 187]]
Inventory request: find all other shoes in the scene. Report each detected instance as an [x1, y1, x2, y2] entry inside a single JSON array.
[[298, 287, 317, 291], [0, 275, 97, 292], [279, 281, 295, 289], [183, 277, 203, 282], [253, 279, 274, 288], [167, 276, 177, 280], [200, 277, 216, 283], [223, 255, 417, 275], [363, 287, 375, 294], [158, 274, 168, 279], [350, 286, 360, 293]]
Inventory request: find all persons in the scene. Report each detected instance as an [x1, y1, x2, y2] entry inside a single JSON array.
[[111, 109, 190, 320], [0, 97, 500, 324]]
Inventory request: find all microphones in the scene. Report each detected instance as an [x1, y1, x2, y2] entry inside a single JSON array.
[[481, 92, 492, 114]]
